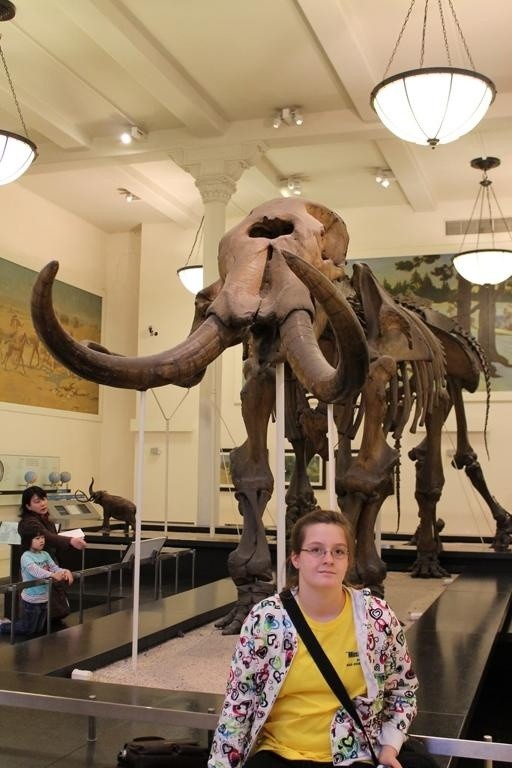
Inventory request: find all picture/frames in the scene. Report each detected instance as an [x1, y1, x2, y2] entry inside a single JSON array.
[[219, 446, 326, 491]]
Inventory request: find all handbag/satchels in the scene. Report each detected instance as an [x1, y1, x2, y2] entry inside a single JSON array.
[[113, 736, 209, 768], [388, 735, 437, 767]]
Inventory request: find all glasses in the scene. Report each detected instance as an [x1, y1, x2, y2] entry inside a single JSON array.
[[299, 546, 350, 560]]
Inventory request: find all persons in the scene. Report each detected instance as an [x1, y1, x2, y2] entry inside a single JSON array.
[[206, 509, 420, 768], [16, 485, 88, 631], [0, 517, 74, 638]]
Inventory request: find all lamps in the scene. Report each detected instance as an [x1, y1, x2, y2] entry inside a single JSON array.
[[117, 187, 140, 203], [451, 157, 512, 287], [279, 171, 309, 197], [177, 216, 204, 295], [270, 105, 305, 129], [370, 0, 496, 152], [0, 0, 39, 186], [371, 168, 396, 189], [119, 126, 145, 144]]
